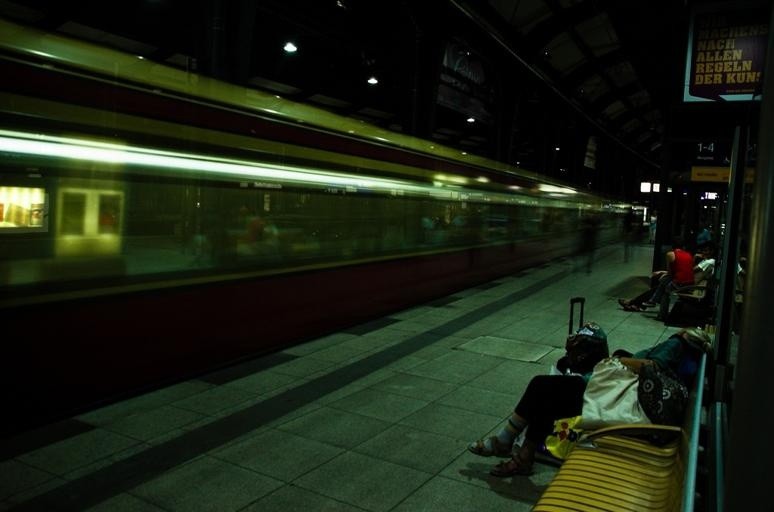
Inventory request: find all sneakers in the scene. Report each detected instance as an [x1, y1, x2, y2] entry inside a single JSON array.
[[618, 298, 656, 311]]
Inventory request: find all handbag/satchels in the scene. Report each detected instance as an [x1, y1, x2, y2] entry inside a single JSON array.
[[544, 356, 689, 459], [567, 322, 609, 370]]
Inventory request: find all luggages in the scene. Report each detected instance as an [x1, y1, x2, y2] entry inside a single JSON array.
[[538, 297, 606, 466]]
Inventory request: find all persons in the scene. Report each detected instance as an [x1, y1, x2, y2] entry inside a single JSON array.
[[467, 325, 711, 478], [618, 238, 718, 321]]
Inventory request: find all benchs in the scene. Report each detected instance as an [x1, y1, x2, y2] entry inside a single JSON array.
[[670, 265, 714, 302], [530, 352, 707, 512]]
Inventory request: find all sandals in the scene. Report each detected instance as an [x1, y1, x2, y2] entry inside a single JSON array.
[[490, 457, 533, 477], [468, 436, 513, 458]]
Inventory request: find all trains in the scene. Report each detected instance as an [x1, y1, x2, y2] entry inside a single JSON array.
[[1, 21, 652, 413]]
